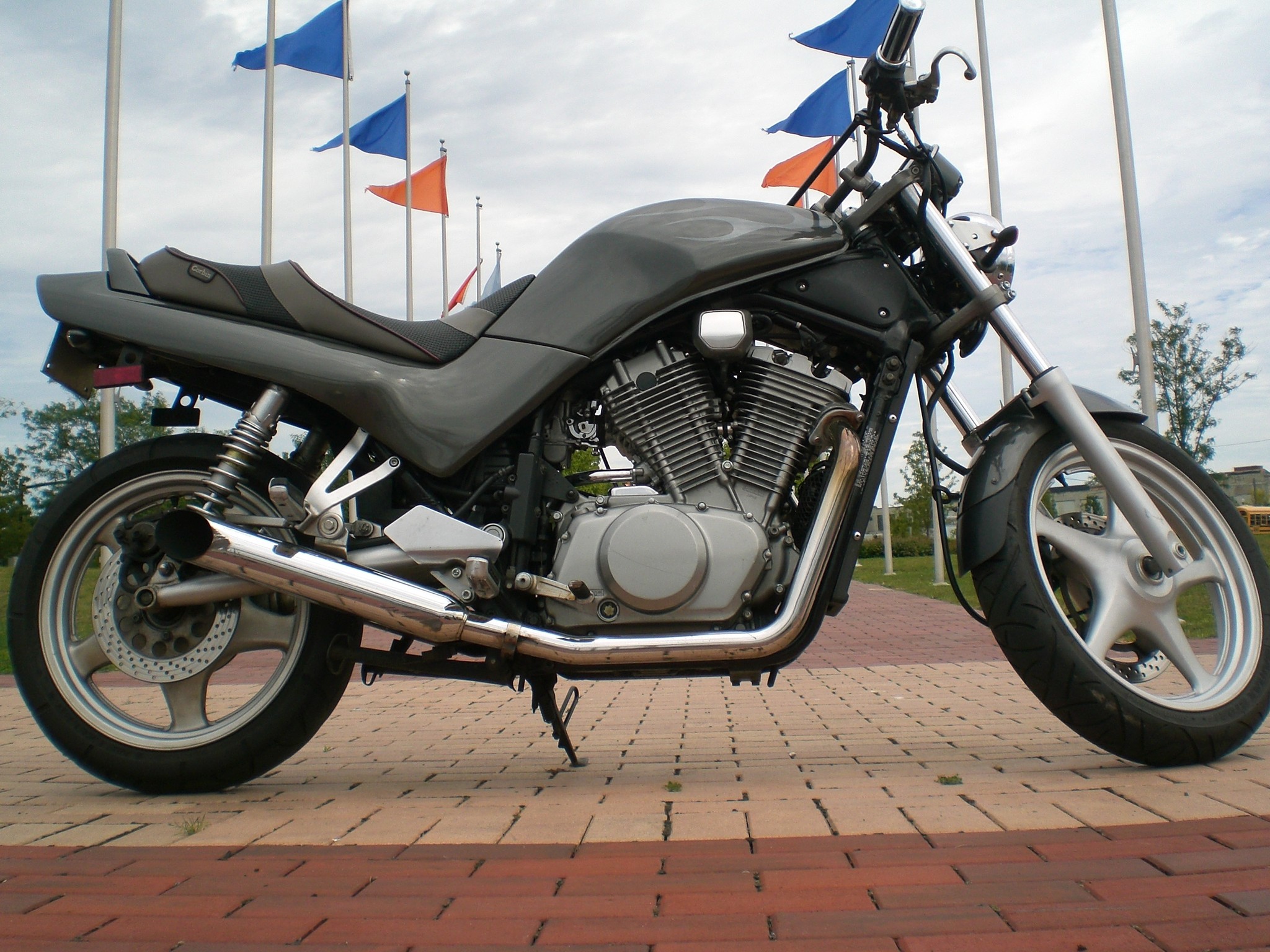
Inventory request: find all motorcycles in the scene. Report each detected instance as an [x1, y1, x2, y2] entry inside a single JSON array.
[[5, 0, 1270, 797]]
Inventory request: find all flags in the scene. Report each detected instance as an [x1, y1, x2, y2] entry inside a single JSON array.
[[463, 253, 502, 308], [761, 67, 855, 139], [793, 194, 805, 208], [364, 155, 449, 218], [440, 260, 483, 318], [230, 0, 350, 82], [788, 0, 899, 58], [310, 93, 407, 161], [761, 136, 838, 196]]
